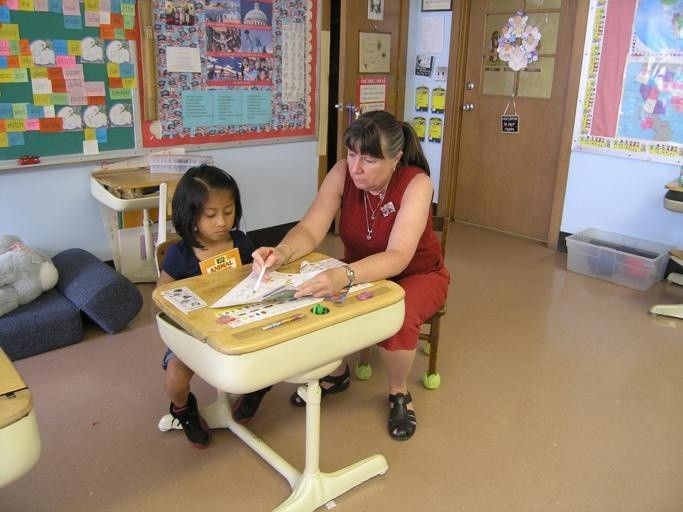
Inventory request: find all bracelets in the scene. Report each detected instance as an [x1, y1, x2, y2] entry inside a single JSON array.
[[275, 243, 294, 263]]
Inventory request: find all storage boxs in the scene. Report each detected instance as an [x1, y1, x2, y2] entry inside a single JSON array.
[[565, 226, 672, 292]]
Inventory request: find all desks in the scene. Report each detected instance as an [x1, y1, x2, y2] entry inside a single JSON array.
[[152, 253, 407, 512], [87, 166, 185, 278]]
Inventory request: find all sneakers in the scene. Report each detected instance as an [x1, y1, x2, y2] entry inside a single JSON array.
[[232, 386, 271, 424], [169, 391, 212, 448]]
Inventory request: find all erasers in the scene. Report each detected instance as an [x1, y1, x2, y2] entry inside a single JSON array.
[[358, 291, 374, 299]]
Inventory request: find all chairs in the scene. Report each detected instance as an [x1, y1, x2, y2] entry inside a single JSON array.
[[351, 212, 451, 391]]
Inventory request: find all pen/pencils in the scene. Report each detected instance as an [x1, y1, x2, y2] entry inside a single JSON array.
[[260, 316, 307, 331], [253, 250, 273, 293]]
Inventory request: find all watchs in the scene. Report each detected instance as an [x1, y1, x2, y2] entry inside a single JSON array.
[[342, 263, 356, 287]]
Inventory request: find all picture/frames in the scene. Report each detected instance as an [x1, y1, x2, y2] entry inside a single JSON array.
[[420, 0, 453, 12]]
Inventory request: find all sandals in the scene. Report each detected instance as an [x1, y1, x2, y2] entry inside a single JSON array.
[[388, 386, 417, 441], [291, 364, 350, 407]]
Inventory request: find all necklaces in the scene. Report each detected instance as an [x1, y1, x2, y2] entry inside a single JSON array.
[[366, 185, 388, 221], [362, 189, 384, 240]]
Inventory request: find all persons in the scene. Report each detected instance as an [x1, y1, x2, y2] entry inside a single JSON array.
[[251, 111, 452, 440], [154, 164, 266, 450], [206, 10, 276, 84]]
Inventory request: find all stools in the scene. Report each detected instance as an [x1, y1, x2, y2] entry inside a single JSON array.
[[0, 247, 144, 360]]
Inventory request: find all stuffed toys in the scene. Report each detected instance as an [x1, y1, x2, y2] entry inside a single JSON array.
[[0, 233, 58, 317]]
